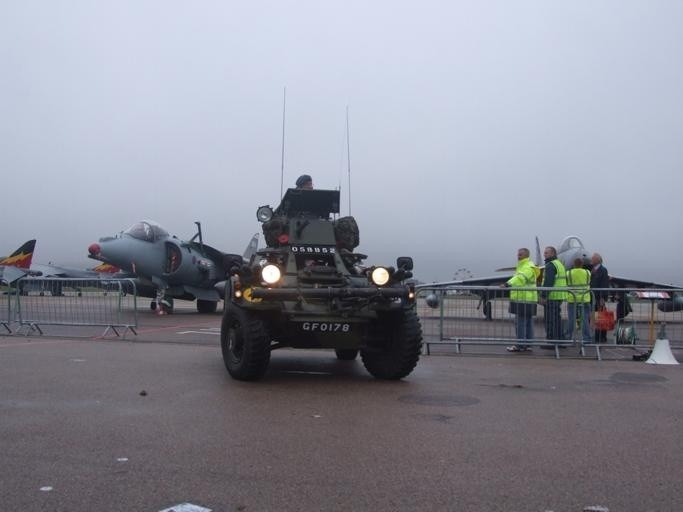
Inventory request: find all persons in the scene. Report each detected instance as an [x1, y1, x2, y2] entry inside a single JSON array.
[[296, 174, 314, 190], [565, 257, 594, 345], [498, 247, 542, 352], [537, 246, 568, 351], [588, 252, 610, 344]]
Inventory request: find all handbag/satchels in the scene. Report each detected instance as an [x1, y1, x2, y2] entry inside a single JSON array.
[[590, 304, 615, 331]]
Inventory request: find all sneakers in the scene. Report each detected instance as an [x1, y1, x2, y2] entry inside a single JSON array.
[[506, 343, 533, 352]]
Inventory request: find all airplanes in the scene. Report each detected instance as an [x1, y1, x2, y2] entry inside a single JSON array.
[[414, 236, 683, 344], [0, 221, 260, 315]]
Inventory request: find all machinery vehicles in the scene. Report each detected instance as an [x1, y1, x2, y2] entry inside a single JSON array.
[[215, 188, 423, 381]]
